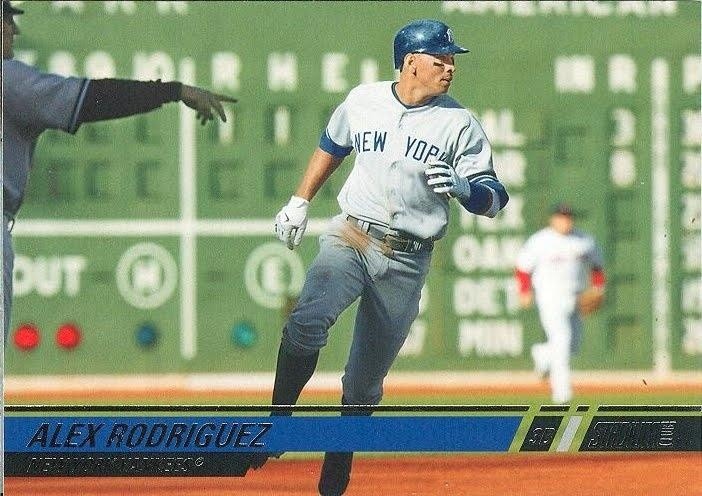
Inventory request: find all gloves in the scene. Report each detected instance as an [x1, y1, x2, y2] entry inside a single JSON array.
[[424, 161, 470, 201], [181, 85, 236, 126], [274, 195, 309, 250]]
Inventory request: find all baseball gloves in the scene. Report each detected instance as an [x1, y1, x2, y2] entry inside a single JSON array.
[[576, 288, 603, 316]]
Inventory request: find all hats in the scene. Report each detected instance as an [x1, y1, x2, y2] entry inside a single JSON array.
[[0, 1, 26, 13]]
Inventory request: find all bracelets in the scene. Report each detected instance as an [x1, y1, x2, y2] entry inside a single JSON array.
[[163, 82, 180, 105], [460, 182, 489, 213]]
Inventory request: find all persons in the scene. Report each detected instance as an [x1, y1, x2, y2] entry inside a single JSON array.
[[3, 0, 239, 347], [514, 202, 605, 404], [251, 19, 509, 496]]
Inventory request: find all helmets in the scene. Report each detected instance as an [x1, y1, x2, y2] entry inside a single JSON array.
[[393, 20, 469, 70]]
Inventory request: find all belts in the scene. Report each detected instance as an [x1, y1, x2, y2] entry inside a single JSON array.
[[346, 215, 432, 253]]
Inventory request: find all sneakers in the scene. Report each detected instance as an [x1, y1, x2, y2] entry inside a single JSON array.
[[318, 451, 353, 495]]
